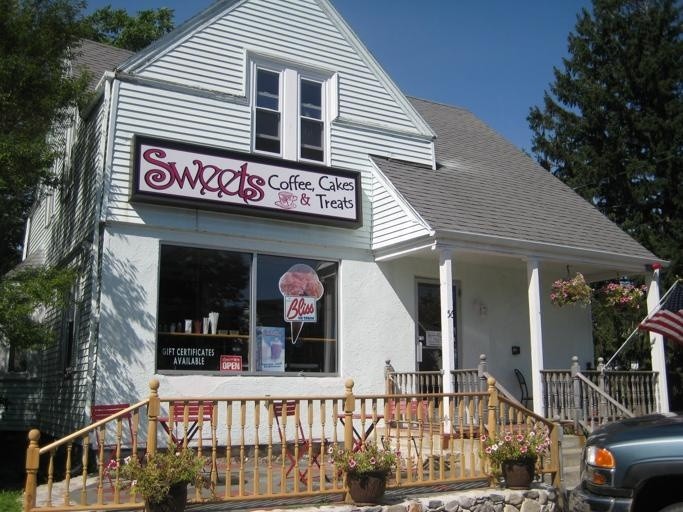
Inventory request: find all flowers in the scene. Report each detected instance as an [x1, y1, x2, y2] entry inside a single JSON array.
[[548, 273, 649, 312], [479, 418, 555, 471]]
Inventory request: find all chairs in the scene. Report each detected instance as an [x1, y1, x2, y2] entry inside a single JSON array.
[[513, 368, 533, 409]]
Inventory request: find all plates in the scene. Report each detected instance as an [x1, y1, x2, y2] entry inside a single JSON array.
[[275, 201, 297, 210], [278, 263, 324, 302]]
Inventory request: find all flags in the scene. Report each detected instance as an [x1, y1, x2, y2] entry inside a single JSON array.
[[636, 277, 682, 347]]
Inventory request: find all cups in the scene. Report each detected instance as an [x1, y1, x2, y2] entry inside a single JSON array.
[[271, 342, 282, 360], [185, 319, 192, 334], [218, 330, 228, 335], [230, 330, 239, 336], [202, 318, 210, 335], [209, 313, 219, 335], [278, 192, 298, 206]]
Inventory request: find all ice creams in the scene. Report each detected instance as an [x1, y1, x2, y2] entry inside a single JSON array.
[[279, 263, 325, 344]]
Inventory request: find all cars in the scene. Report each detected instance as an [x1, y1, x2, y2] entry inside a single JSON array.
[[578, 410, 682, 512]]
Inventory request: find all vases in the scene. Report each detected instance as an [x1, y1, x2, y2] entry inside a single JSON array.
[[144, 479, 192, 511], [346, 472, 387, 505], [501, 455, 536, 490]]
